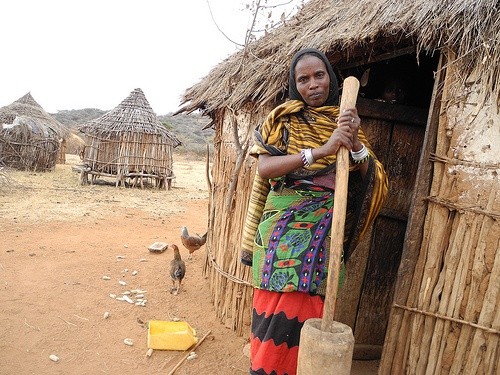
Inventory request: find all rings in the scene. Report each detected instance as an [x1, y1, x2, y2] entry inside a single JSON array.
[[351, 118, 355, 123]]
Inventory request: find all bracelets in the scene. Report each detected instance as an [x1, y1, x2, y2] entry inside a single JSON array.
[[353, 154, 369, 164], [301, 150, 311, 168], [304, 148, 316, 165], [351, 143, 368, 160]]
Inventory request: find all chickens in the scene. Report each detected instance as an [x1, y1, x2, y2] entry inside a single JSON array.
[[180, 226, 208, 259], [169, 245, 186, 295]]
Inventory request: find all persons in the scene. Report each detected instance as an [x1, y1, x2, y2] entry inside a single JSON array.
[[249, 48, 389, 375]]
